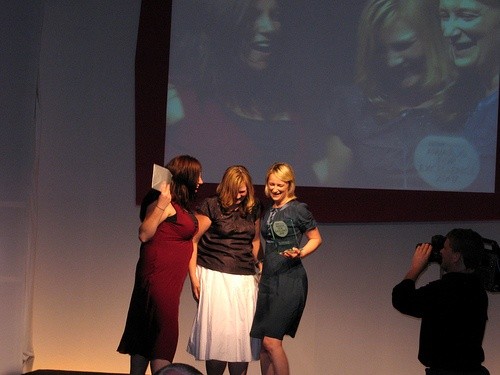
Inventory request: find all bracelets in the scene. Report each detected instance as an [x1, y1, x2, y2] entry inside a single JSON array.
[[156, 205, 165, 211], [255, 260, 259, 264]]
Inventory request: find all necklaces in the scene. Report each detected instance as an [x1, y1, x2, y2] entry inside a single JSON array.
[[282, 199, 288, 204]]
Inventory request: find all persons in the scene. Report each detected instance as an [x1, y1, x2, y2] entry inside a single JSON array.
[[162, 0, 500, 191], [392, 227, 492, 375], [116, 153, 203, 375], [250, 161, 322, 375], [153, 362, 204, 375], [188, 164, 263, 375]]
